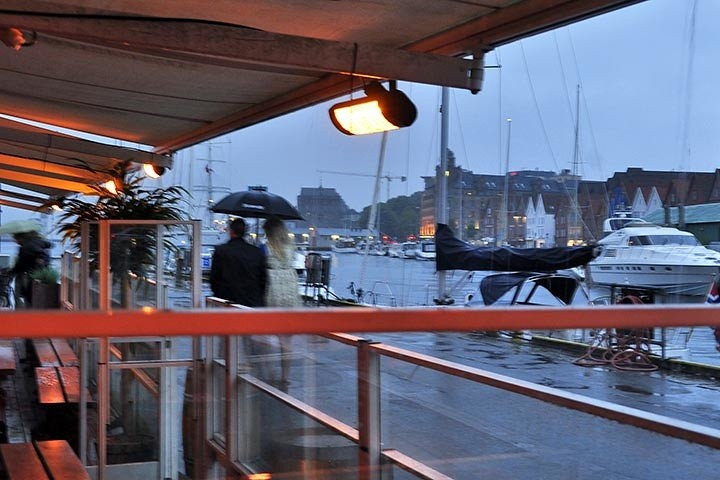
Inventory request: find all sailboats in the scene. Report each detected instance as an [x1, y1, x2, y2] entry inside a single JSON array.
[[0, 80, 587, 308]]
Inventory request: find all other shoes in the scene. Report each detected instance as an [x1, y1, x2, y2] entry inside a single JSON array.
[[281, 380, 290, 386], [19, 357, 32, 364]]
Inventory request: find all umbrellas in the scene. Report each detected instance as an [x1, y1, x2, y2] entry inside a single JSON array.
[[0, 220, 43, 234], [210, 186, 305, 246]]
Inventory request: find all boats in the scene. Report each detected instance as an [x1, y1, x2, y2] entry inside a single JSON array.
[[583, 202, 720, 299]]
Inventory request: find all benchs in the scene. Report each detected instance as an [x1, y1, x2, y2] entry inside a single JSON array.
[[0, 335, 91, 480]]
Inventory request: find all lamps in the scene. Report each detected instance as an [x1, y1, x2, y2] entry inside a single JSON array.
[[329, 80, 417, 136], [144, 163, 164, 178]]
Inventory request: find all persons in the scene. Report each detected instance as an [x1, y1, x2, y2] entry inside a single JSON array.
[[211, 218, 268, 399], [8, 229, 53, 311], [261, 219, 300, 386]]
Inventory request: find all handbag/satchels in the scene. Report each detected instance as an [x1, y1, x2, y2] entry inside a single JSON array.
[[14, 273, 33, 297]]
